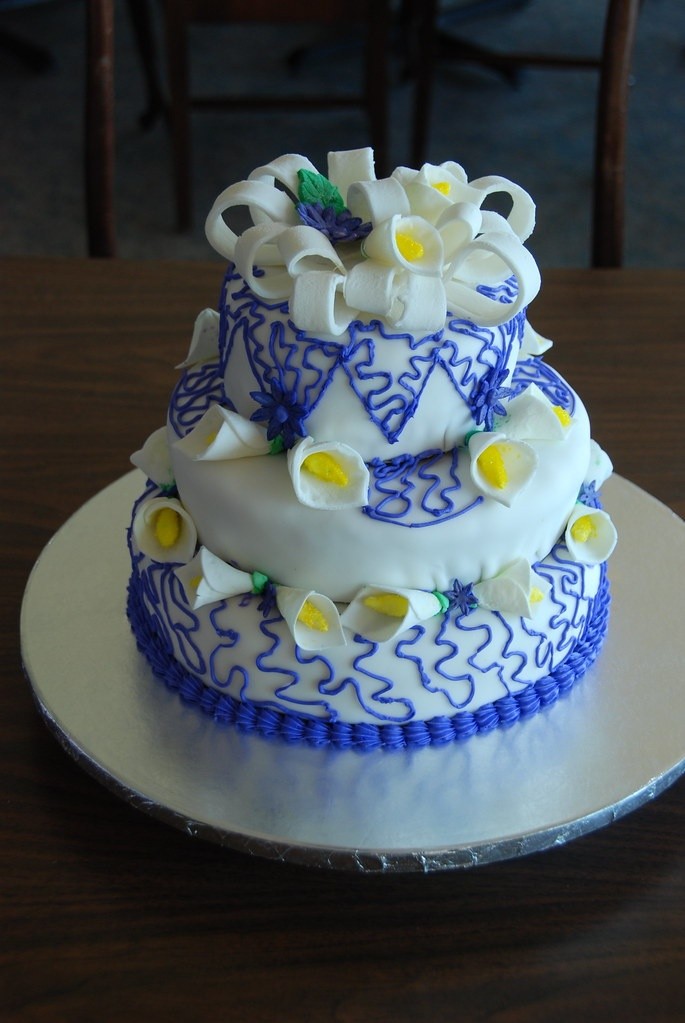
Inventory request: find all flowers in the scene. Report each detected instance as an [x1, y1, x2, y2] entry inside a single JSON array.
[[473, 368, 514, 432], [296, 201, 377, 246], [247, 378, 311, 451], [257, 583, 276, 618], [443, 579, 478, 615], [577, 481, 602, 508]]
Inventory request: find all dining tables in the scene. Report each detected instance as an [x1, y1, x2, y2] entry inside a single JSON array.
[[0, 254, 685, 1023]]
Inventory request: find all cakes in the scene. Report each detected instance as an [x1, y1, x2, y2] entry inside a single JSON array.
[[127, 148, 621, 754]]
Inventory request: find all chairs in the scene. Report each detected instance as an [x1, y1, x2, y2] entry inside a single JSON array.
[[81, 0, 642, 272]]
